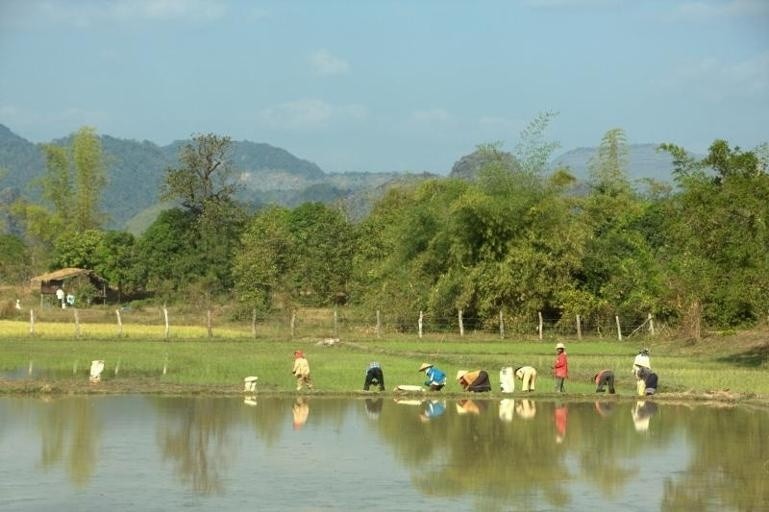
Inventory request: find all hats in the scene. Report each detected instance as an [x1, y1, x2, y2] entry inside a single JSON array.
[[555, 343, 565, 348], [594, 373, 599, 383], [419, 363, 433, 371], [514, 367, 523, 379], [420, 415, 431, 423], [634, 361, 650, 369], [456, 370, 469, 380], [456, 403, 466, 415]]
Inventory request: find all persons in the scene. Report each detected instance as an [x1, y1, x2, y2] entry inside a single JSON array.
[[513, 398, 537, 420], [456, 369, 492, 392], [291, 349, 313, 391], [364, 397, 385, 422], [630, 399, 652, 438], [455, 398, 488, 418], [591, 399, 617, 420], [631, 346, 651, 394], [362, 362, 385, 391], [635, 360, 660, 398], [56, 286, 65, 309], [592, 368, 616, 393], [513, 365, 538, 393], [550, 342, 569, 392], [553, 400, 570, 445], [634, 401, 660, 420], [291, 397, 311, 432], [417, 397, 448, 426], [418, 362, 446, 391]]
